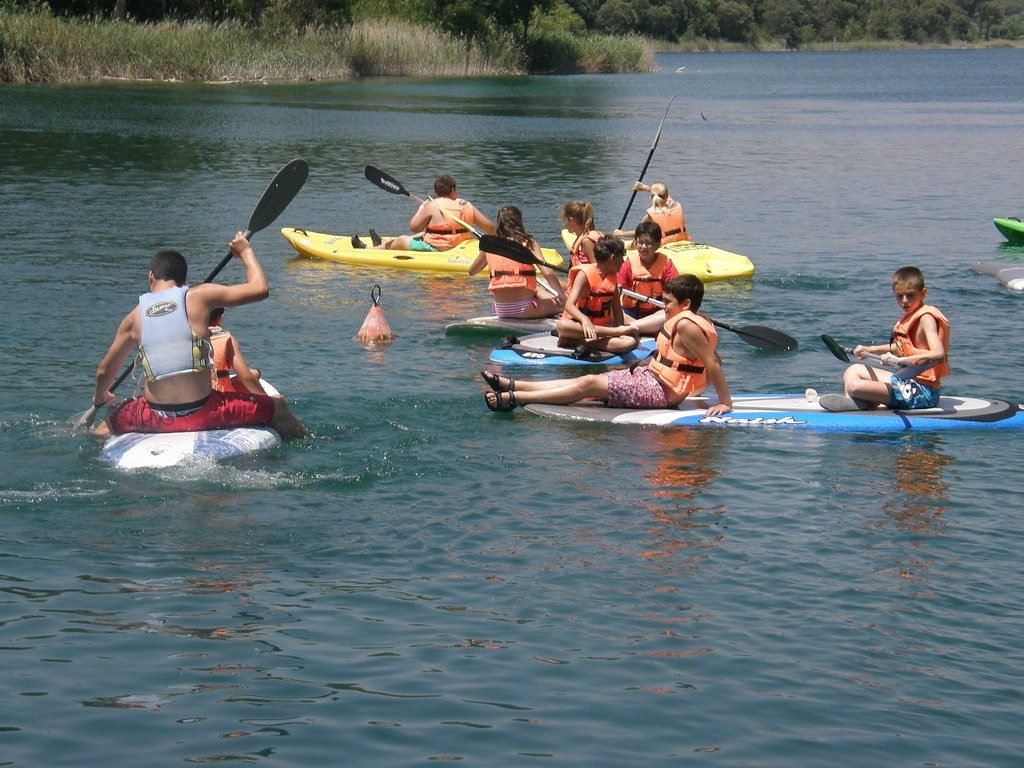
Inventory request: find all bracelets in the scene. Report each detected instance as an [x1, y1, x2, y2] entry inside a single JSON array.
[[720, 400, 732, 408]]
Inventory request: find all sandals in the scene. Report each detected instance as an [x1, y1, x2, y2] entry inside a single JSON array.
[[484, 390, 517, 411], [482, 370, 514, 391]]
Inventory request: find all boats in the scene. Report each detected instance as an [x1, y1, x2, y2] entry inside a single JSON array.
[[280, 226, 565, 274], [993, 216, 1024, 245], [560, 227, 757, 284], [102, 374, 281, 472]]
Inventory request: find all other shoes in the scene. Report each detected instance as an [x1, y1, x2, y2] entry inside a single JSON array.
[[369, 227, 382, 246], [819, 394, 871, 411], [351, 235, 367, 249]]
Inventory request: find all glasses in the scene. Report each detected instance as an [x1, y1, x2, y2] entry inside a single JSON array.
[[614, 248, 626, 256]]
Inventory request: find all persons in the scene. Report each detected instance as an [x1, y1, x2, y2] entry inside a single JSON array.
[[92, 230, 309, 438], [563, 200, 605, 264], [469, 206, 568, 318], [207, 307, 283, 399], [482, 274, 733, 416], [350, 174, 497, 252], [819, 265, 950, 411], [613, 181, 691, 248], [555, 234, 639, 358], [616, 221, 681, 333]]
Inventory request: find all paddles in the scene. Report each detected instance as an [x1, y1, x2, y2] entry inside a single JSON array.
[[819, 332, 946, 380], [362, 163, 485, 242], [616, 92, 677, 231], [478, 233, 801, 353], [66, 157, 312, 430]]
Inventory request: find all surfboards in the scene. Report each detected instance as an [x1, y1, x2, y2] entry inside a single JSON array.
[[444, 314, 658, 367], [522, 391, 1024, 435], [102, 375, 285, 469]]
